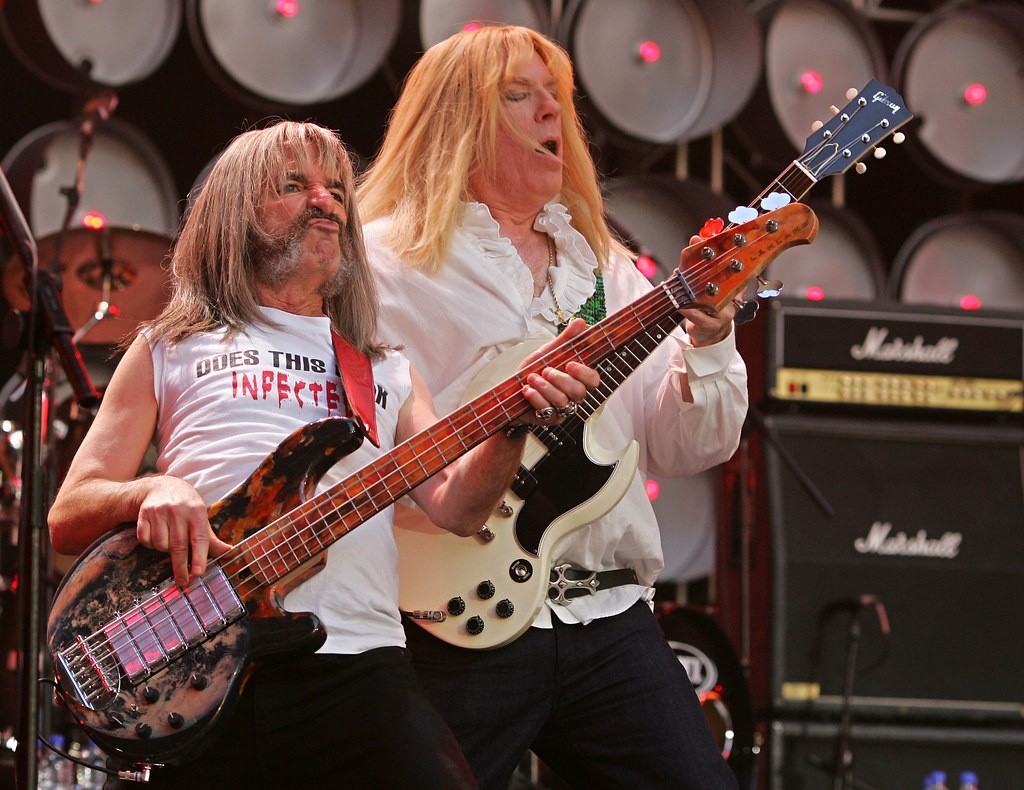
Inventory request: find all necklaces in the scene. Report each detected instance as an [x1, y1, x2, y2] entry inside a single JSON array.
[[546, 230, 568, 326]]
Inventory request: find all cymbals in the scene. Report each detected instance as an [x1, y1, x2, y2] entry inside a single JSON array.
[[0, 222, 180, 346]]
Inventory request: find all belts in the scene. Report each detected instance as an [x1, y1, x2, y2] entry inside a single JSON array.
[[548, 564, 639, 606]]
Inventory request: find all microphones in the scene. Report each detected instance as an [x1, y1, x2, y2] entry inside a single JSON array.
[[78, 86, 118, 137], [823, 593, 876, 616]]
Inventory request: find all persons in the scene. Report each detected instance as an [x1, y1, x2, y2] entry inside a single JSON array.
[[46, 123, 598, 790], [353, 28, 747, 790]]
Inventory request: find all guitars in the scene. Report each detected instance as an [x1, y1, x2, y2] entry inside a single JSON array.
[[43, 189, 821, 767], [393, 71, 919, 653]]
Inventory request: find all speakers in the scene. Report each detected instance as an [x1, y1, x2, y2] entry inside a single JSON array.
[[762, 413, 1024, 730], [761, 723, 1024, 790]]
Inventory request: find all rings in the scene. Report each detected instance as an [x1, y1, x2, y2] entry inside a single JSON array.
[[536, 407, 554, 419], [556, 401, 577, 418]]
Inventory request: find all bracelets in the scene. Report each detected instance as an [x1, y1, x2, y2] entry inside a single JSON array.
[[505, 423, 531, 437]]
